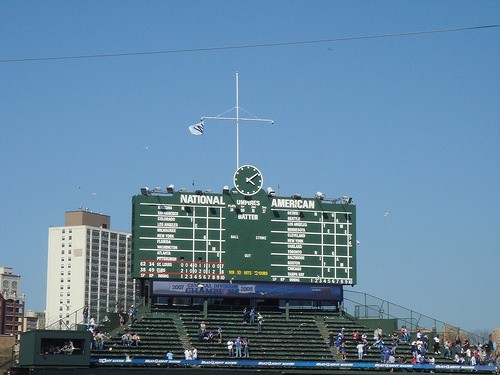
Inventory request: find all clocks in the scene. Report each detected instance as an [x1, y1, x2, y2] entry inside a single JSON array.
[[233, 164, 264, 196]]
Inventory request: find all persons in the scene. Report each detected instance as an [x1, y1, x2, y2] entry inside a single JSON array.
[[118, 313, 124, 327], [49, 340, 74, 354], [122, 332, 139, 346], [185, 347, 197, 361], [243, 307, 262, 332], [90, 316, 96, 327], [330, 326, 500, 375], [129, 305, 136, 321], [166, 350, 174, 360], [225, 337, 249, 357], [93, 331, 109, 341], [198, 321, 223, 343]]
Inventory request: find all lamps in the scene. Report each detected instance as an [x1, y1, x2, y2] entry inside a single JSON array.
[[267, 188, 276, 196], [342, 196, 353, 204], [166, 184, 174, 191], [222, 185, 230, 194], [315, 192, 325, 200], [141, 187, 150, 192]]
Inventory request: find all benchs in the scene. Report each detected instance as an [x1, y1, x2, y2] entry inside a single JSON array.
[[92, 310, 478, 365]]
